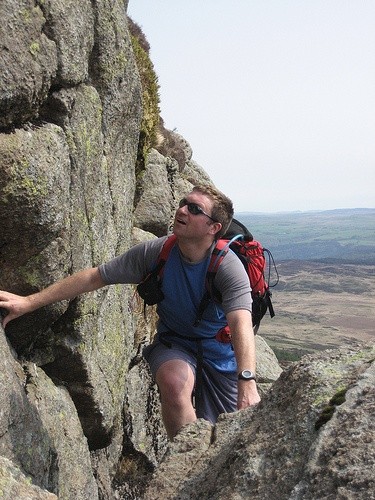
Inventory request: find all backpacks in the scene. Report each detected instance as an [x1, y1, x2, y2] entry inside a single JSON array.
[[149, 224, 279, 343]]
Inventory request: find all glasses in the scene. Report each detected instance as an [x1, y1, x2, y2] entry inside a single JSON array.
[[177, 198, 219, 225]]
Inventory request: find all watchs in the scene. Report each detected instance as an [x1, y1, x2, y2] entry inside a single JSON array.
[[237, 369, 257, 382]]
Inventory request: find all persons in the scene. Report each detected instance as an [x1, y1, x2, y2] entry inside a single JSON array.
[[0, 184, 263, 441]]
